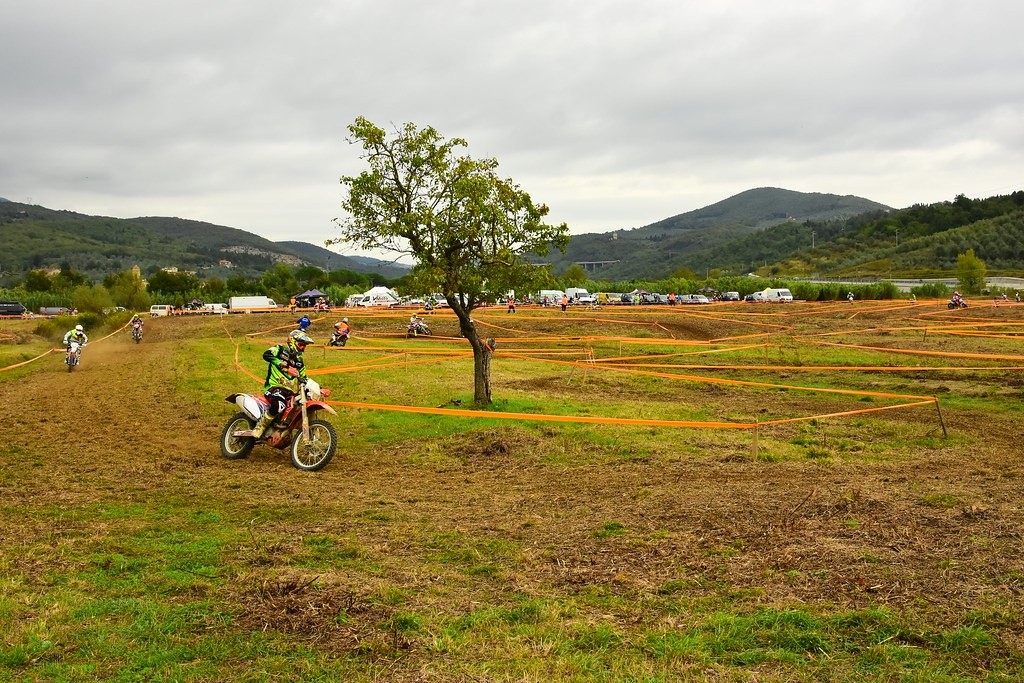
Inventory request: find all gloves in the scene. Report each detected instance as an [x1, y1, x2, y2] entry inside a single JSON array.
[[287, 366, 298, 377], [320, 388, 331, 397], [63, 341, 68, 344]]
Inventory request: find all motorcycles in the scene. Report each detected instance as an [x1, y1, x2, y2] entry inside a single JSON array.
[[131, 322, 143, 343], [220, 365, 339, 472], [948, 296, 968, 308], [65, 341, 83, 372], [326, 326, 350, 347], [313, 302, 330, 313], [584, 302, 602, 312], [848, 295, 854, 305], [406, 318, 432, 336]]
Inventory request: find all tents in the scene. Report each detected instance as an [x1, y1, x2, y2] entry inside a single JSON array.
[[292, 289, 328, 309]]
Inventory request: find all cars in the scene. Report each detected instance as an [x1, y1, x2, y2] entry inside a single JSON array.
[[439, 295, 468, 307], [621, 293, 692, 304], [720, 292, 763, 301]]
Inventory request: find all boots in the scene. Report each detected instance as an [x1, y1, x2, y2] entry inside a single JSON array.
[[251, 411, 275, 439], [331, 335, 336, 343], [414, 330, 417, 336]]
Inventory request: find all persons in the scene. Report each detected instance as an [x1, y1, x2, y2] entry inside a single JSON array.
[[296, 313, 311, 331], [63, 324, 88, 366], [251, 329, 315, 440], [43, 306, 78, 316], [289, 297, 358, 314], [507, 294, 722, 312], [331, 318, 350, 344], [130, 314, 144, 340], [166, 297, 207, 317], [407, 300, 433, 336]]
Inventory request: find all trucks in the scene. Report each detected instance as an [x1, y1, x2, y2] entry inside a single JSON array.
[[357, 294, 398, 306], [229, 296, 278, 313]]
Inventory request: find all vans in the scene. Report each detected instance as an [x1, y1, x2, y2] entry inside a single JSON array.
[[688, 295, 709, 304], [762, 288, 793, 303], [149, 303, 229, 316], [565, 288, 596, 304], [103, 307, 126, 315], [541, 290, 570, 305], [598, 293, 622, 305]]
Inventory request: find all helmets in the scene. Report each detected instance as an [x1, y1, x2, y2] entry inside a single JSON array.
[[76, 325, 83, 331], [412, 313, 417, 316], [342, 318, 349, 322], [288, 330, 315, 355], [134, 315, 138, 319], [304, 313, 309, 317]]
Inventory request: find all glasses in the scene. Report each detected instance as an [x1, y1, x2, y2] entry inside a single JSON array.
[[295, 341, 306, 348]]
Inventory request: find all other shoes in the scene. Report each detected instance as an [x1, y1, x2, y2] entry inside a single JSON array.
[[76, 361, 79, 365], [65, 359, 68, 364]]
